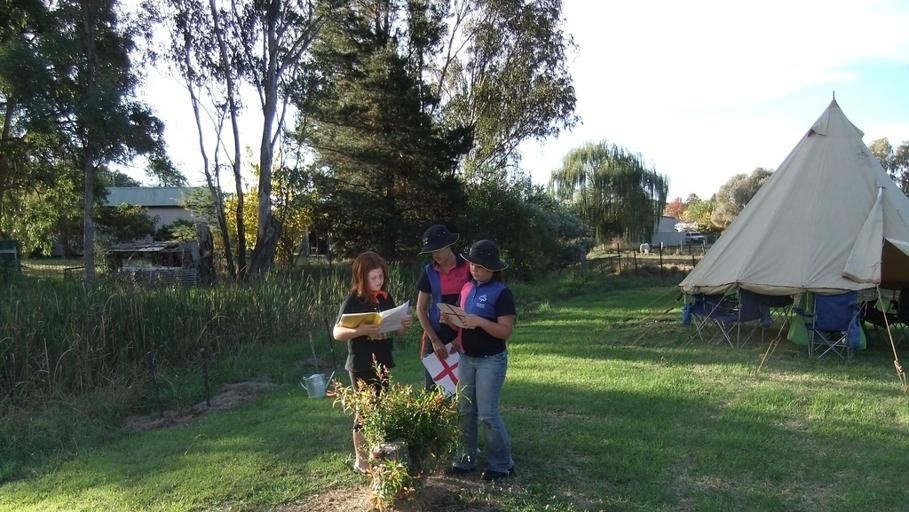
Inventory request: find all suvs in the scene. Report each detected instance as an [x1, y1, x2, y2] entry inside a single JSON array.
[[686, 231, 705, 244]]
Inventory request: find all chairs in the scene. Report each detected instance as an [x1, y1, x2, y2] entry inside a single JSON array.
[[683, 287, 909, 363]]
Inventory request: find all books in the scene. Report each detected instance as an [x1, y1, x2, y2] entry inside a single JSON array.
[[335, 300, 409, 335]]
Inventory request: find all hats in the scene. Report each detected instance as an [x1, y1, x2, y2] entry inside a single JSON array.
[[459, 239, 509, 272], [416, 224, 460, 255]]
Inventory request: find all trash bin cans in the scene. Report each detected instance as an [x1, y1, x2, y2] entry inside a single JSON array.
[[0, 240, 21, 270]]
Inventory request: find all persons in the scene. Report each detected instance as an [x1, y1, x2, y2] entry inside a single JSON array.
[[331, 252, 413, 474], [415, 225, 472, 407], [437, 238, 519, 481]]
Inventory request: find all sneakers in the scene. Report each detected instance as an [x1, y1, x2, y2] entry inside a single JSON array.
[[445, 466, 476, 475], [480, 466, 514, 480]]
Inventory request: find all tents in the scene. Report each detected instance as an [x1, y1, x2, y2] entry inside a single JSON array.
[[674, 91, 909, 382]]
